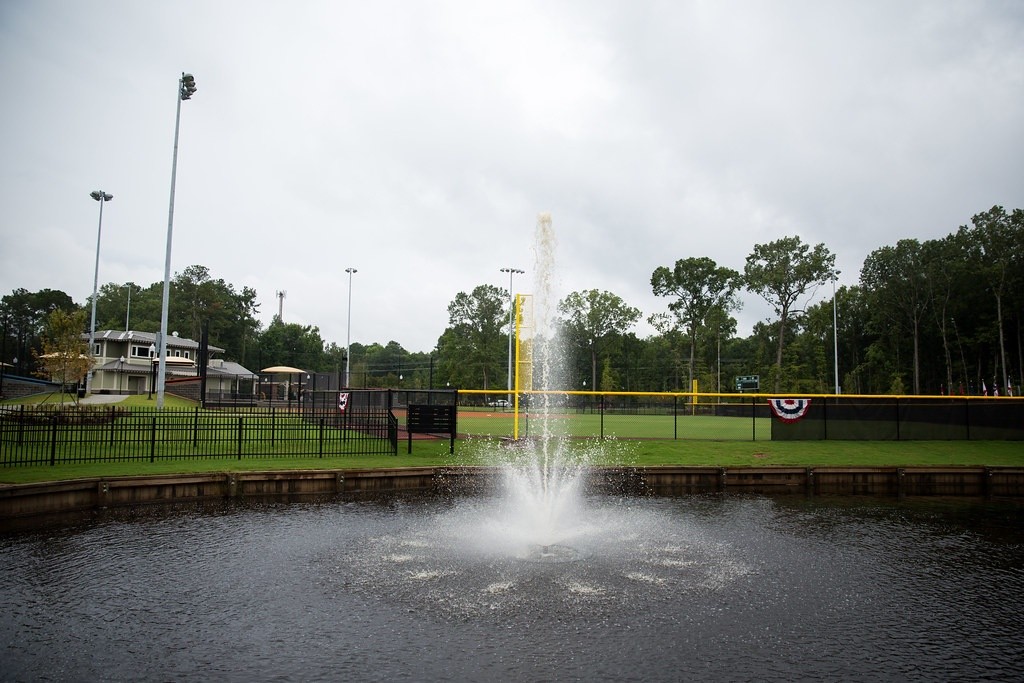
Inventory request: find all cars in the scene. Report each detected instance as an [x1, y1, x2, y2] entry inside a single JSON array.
[[488, 400, 513, 407]]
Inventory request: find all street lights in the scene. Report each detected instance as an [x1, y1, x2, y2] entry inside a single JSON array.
[[147, 343, 156, 400], [827, 271, 838, 396], [344, 268, 359, 389], [156, 71, 197, 398], [84, 190, 114, 397], [498, 267, 526, 410], [120, 356, 125, 394]]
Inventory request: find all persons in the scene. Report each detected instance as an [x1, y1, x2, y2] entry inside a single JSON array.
[[276, 386, 282, 400], [295, 385, 306, 399], [260, 392, 266, 400]]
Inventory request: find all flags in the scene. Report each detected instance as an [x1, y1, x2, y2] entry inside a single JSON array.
[[941, 379, 976, 396], [982, 379, 987, 396], [993, 377, 998, 396], [1007, 376, 1012, 396]]
